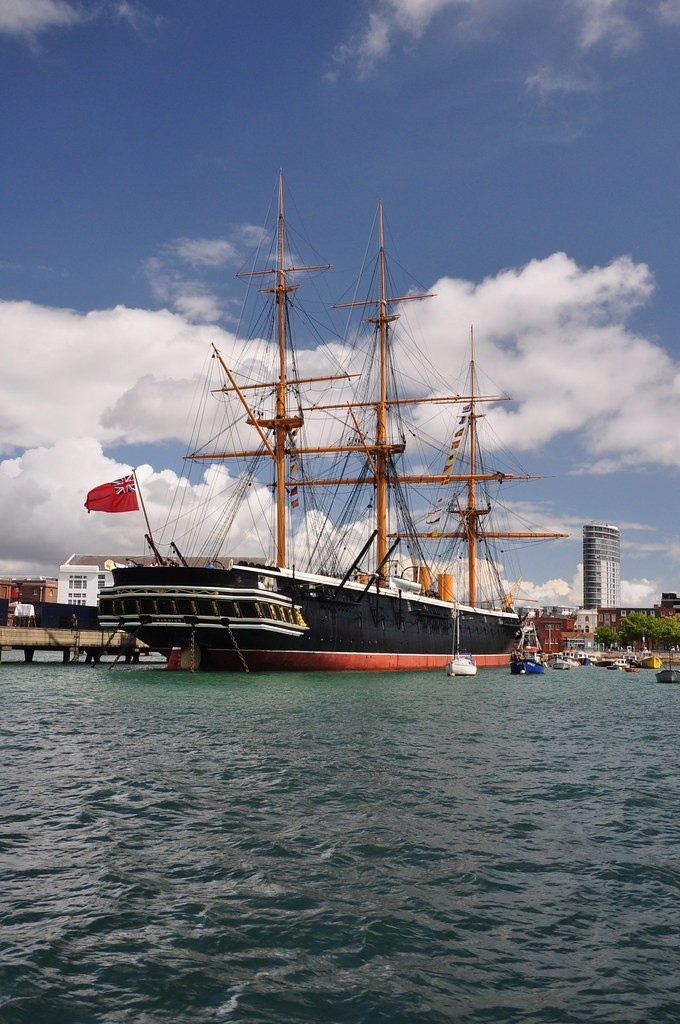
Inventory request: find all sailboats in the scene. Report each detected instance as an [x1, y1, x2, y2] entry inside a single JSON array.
[[444, 599, 478, 677]]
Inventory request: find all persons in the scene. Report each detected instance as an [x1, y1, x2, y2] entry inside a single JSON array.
[[71, 614, 77, 633], [169, 559, 179, 568], [126, 560, 136, 568], [539, 656, 548, 668], [510, 649, 526, 663], [207, 561, 214, 568], [149, 559, 159, 568]]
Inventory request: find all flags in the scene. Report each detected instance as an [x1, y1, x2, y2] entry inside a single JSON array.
[[84, 473, 139, 514]]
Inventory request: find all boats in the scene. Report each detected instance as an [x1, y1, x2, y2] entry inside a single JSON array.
[[655, 648, 680, 683], [507, 621, 665, 677], [91, 170, 568, 674]]
